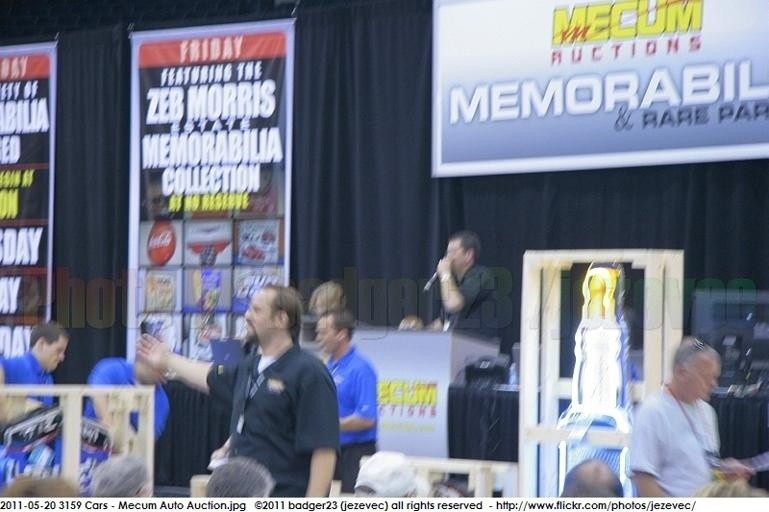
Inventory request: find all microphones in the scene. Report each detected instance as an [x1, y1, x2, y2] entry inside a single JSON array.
[[422, 258, 445, 296]]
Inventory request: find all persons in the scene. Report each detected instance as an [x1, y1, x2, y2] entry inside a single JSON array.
[[428, 230, 503, 348], [628, 336, 758, 497], [696, 478, 769, 497], [561, 457, 623, 498]]
